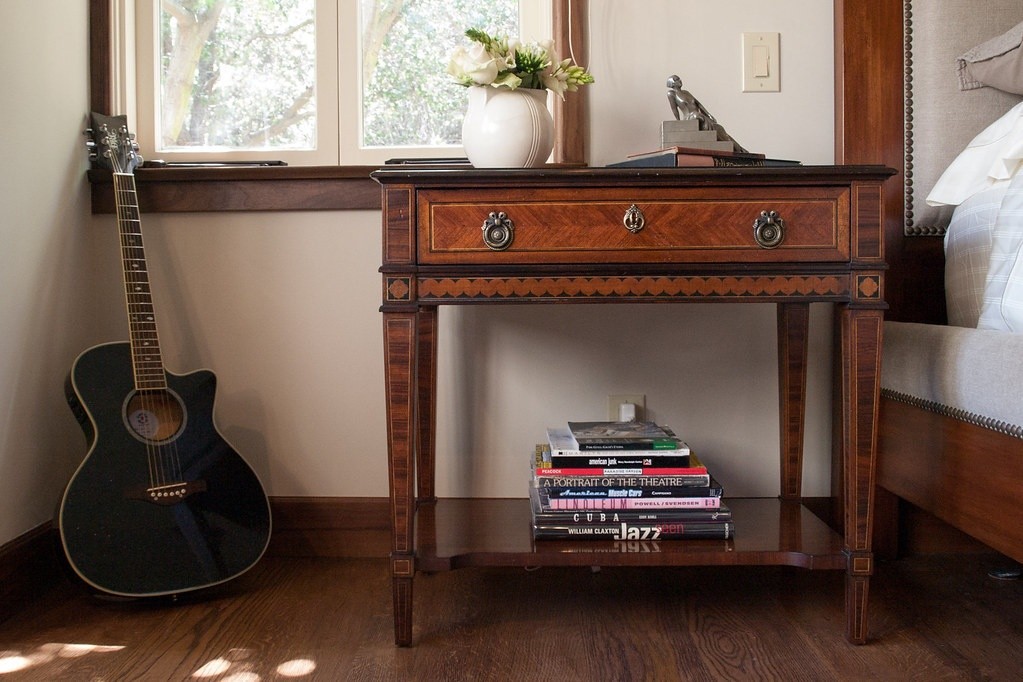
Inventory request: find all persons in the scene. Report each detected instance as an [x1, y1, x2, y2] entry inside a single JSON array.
[[666, 75, 749, 152]]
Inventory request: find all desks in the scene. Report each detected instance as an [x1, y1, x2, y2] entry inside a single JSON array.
[[369, 157, 899, 649]]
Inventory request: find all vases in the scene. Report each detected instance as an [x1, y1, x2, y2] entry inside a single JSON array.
[[461, 87, 554, 169]]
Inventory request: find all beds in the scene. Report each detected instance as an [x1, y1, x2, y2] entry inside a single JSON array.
[[833, 0, 1022, 564]]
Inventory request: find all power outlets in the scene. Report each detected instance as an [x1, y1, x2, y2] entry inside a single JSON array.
[[606, 393, 646, 423]]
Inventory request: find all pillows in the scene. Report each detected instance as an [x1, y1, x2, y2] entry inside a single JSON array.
[[925, 101, 1023, 207], [955, 19, 1023, 98]]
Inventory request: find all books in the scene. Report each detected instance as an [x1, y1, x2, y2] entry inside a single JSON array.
[[527, 420, 734, 542], [606, 145, 803, 168]]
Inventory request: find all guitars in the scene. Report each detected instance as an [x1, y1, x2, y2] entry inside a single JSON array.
[[50, 106, 280, 612]]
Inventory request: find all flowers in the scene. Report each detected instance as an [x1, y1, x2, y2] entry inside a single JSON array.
[[444, 27, 597, 102]]
